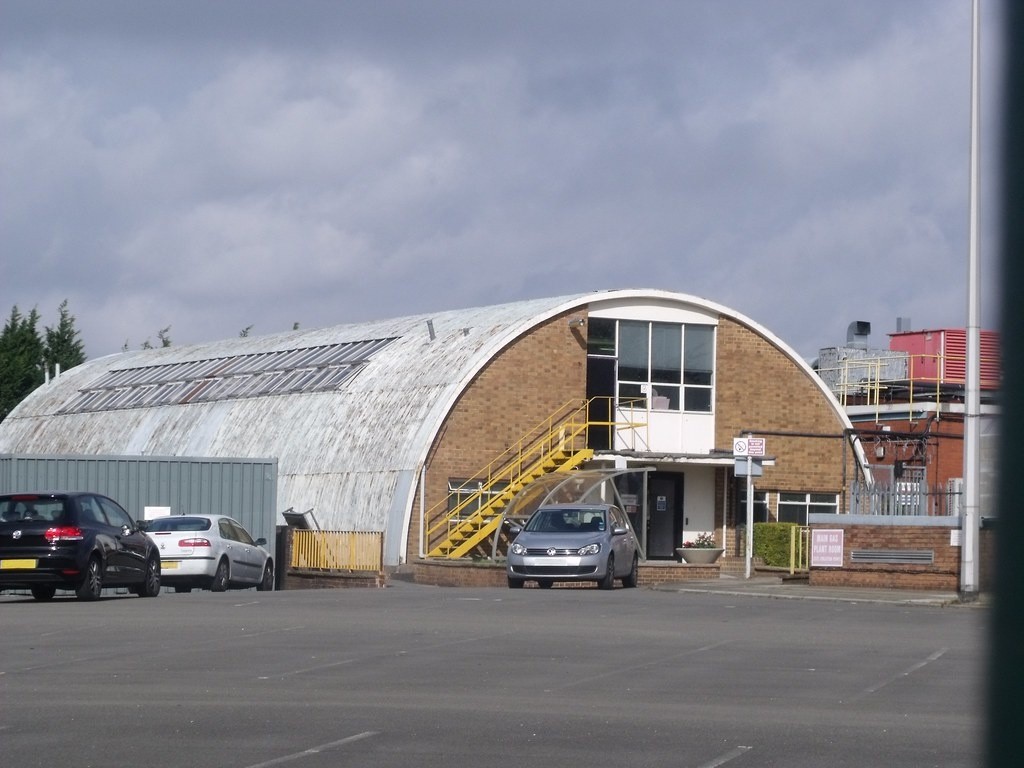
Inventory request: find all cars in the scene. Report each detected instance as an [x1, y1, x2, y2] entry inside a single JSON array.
[[0, 490, 161, 602], [142, 513, 274, 593], [506, 502, 639, 590]]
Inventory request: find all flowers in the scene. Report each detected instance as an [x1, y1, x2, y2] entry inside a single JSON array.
[[682, 533, 718, 549]]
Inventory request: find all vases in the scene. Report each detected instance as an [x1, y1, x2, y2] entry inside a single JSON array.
[[675, 546, 724, 564]]
